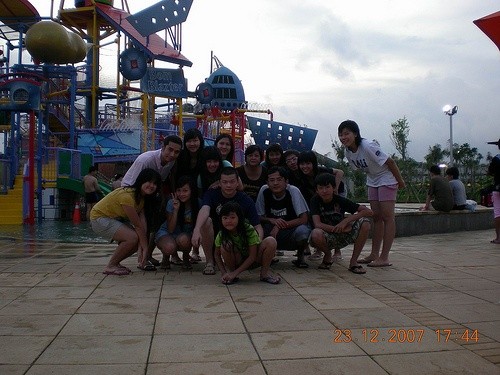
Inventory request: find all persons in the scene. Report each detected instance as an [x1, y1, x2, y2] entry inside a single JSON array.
[[259, 144, 290, 256], [445, 167, 468, 211], [89, 167, 163, 275], [215, 202, 281, 285], [192, 167, 264, 274], [213, 135, 236, 165], [237, 145, 280, 266], [156, 175, 202, 270], [122, 134, 185, 272], [283, 146, 326, 259], [164, 129, 208, 263], [338, 120, 406, 267], [308, 173, 374, 273], [292, 150, 349, 262], [1, 59, 8, 98], [198, 144, 245, 270], [487, 138, 500, 244], [255, 166, 311, 267], [415, 164, 455, 211], [113, 174, 123, 190], [82, 166, 101, 223]]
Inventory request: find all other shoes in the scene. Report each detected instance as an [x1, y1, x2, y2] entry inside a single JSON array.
[[137, 250, 201, 270], [272, 248, 345, 261], [490, 239, 500, 244]]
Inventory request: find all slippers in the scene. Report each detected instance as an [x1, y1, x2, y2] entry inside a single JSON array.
[[102, 265, 130, 275], [358, 255, 393, 267], [319, 259, 332, 270], [348, 264, 367, 274], [292, 259, 309, 268]]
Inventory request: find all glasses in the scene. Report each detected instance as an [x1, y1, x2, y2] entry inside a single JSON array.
[[286, 157, 297, 165]]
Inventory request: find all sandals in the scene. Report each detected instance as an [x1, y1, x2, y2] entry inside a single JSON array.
[[259, 272, 280, 284], [203, 262, 215, 275], [221, 271, 233, 284]]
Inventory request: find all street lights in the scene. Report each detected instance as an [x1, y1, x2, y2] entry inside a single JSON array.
[[443, 105, 459, 152]]
